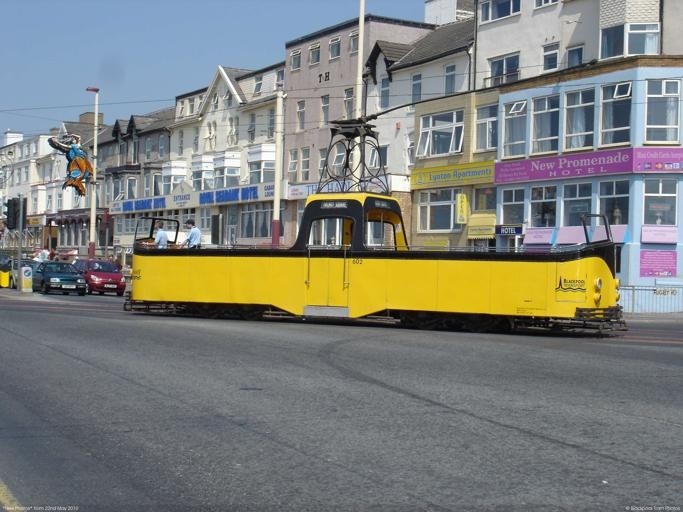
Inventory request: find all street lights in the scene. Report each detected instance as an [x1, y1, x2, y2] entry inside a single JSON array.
[[48, 87, 100, 257]]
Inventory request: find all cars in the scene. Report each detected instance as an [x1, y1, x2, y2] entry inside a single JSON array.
[[33, 262, 89, 295], [75, 259, 126, 296], [0, 259, 44, 290]]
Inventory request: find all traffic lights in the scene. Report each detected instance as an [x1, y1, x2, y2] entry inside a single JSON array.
[[3, 199, 16, 229]]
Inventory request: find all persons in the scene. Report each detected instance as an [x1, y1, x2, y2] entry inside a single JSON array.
[[142, 221, 168, 249], [177, 219, 201, 249]]
